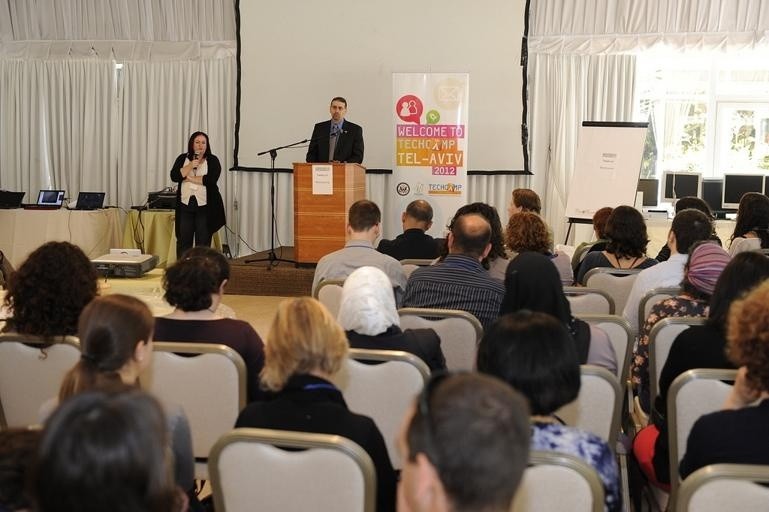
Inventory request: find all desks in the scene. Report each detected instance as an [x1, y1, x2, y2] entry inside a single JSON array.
[[640, 196, 738, 244], [0, 208, 223, 273]]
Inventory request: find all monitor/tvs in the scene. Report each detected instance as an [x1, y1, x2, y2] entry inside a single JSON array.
[[634, 173, 765, 219]]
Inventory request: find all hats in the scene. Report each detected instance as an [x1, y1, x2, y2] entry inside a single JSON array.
[[688, 243, 733, 297]]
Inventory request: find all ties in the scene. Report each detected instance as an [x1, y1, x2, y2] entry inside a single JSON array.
[[329, 124, 338, 161]]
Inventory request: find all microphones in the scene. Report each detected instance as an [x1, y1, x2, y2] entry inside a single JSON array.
[[331, 128, 344, 137], [194, 151, 199, 170]]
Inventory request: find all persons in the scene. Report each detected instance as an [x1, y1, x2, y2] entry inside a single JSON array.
[[169, 130, 226, 262], [306, 96, 364, 165]]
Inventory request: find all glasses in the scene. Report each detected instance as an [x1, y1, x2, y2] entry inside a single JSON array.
[[415, 367, 475, 468]]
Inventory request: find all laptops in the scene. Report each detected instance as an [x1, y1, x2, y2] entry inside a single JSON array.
[[69, 191, 105, 210], [24, 189, 65, 209], [0, 191, 26, 210]]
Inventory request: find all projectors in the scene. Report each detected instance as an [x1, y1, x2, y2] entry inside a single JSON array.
[[89, 249, 160, 278]]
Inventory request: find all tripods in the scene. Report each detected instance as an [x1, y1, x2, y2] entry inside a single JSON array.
[[245, 133, 324, 266]]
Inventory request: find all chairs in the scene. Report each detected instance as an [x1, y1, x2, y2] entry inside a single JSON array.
[[294, 259, 769, 511], [1, 330, 248, 511], [207, 425, 377, 511]]
